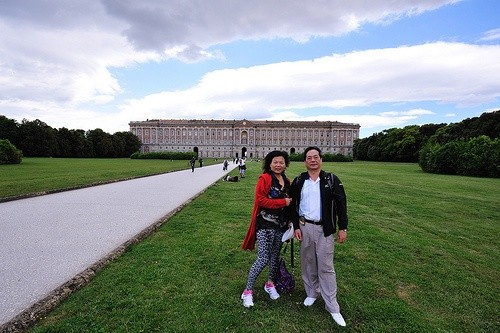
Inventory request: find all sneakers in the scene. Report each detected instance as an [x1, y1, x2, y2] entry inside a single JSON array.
[[303, 296, 317, 307], [264, 282, 280, 301], [241, 289, 254, 309], [330, 309, 347, 326]]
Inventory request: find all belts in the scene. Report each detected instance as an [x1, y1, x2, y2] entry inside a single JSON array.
[[305, 219, 320, 225]]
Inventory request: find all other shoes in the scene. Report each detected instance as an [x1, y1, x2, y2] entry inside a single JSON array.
[[240, 176, 245, 178]]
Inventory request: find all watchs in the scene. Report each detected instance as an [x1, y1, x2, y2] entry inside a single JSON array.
[[343, 228, 346, 231]]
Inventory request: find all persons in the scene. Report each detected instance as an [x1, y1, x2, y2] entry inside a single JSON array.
[[288, 146, 348, 327], [198, 155, 203, 168], [190, 157, 196, 172], [241, 150, 292, 309], [223, 156, 247, 182]]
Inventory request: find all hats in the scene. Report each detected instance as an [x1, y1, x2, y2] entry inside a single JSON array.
[[281, 222, 295, 243]]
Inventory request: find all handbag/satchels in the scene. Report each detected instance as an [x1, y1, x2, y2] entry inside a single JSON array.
[[274, 259, 295, 295]]
